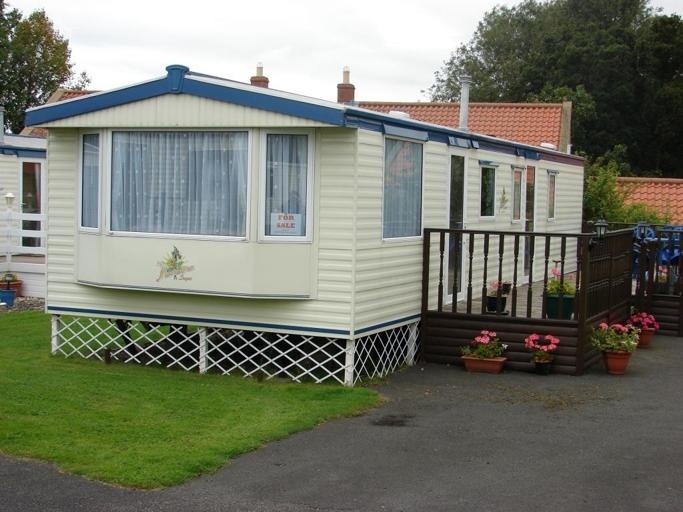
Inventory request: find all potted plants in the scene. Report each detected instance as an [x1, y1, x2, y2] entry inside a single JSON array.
[[0, 273, 22, 296]]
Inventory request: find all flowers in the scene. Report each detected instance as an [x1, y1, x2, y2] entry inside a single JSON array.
[[594, 311, 661, 351], [488, 281, 503, 296], [547, 258, 577, 297], [461, 329, 560, 359]]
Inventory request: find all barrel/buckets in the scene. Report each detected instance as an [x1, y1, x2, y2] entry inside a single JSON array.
[[0, 289, 16, 306]]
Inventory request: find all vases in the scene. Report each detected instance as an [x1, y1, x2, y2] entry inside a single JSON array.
[[484, 295, 507, 311], [605, 348, 630, 375], [546, 295, 573, 319], [462, 354, 553, 376]]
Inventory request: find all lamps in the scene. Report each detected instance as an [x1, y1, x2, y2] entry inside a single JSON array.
[[587, 216, 609, 249], [3, 192, 15, 212]]
